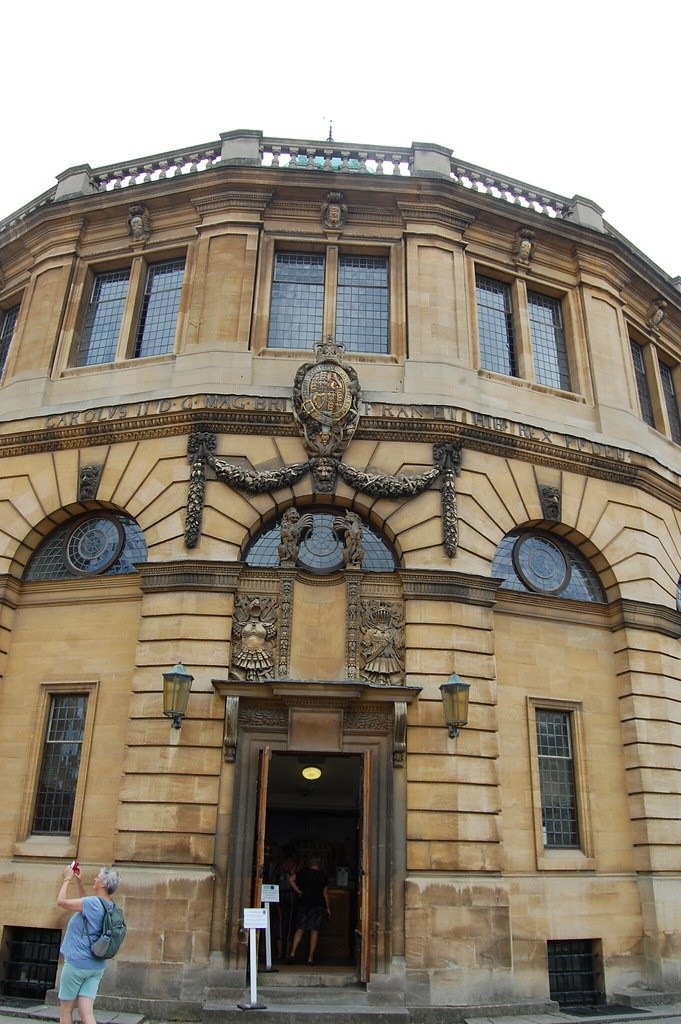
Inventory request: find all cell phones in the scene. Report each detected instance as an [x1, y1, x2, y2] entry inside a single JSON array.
[[72, 861, 79, 873]]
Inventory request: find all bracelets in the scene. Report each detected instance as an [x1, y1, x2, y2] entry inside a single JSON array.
[[64, 879, 70, 882]]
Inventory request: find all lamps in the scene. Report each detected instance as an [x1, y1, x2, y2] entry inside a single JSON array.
[[439, 671, 470, 739], [298, 754, 325, 781], [162, 661, 193, 728]]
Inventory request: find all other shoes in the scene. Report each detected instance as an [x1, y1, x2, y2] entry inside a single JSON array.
[[307, 962, 314, 966], [288, 956, 294, 965]]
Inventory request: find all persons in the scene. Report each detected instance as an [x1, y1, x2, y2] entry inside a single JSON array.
[[271, 845, 331, 966], [57, 866, 120, 1024]]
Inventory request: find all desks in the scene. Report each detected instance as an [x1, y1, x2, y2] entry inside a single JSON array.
[[317, 881, 355, 966]]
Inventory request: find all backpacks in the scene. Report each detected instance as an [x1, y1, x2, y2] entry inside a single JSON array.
[[84, 897, 126, 959]]
